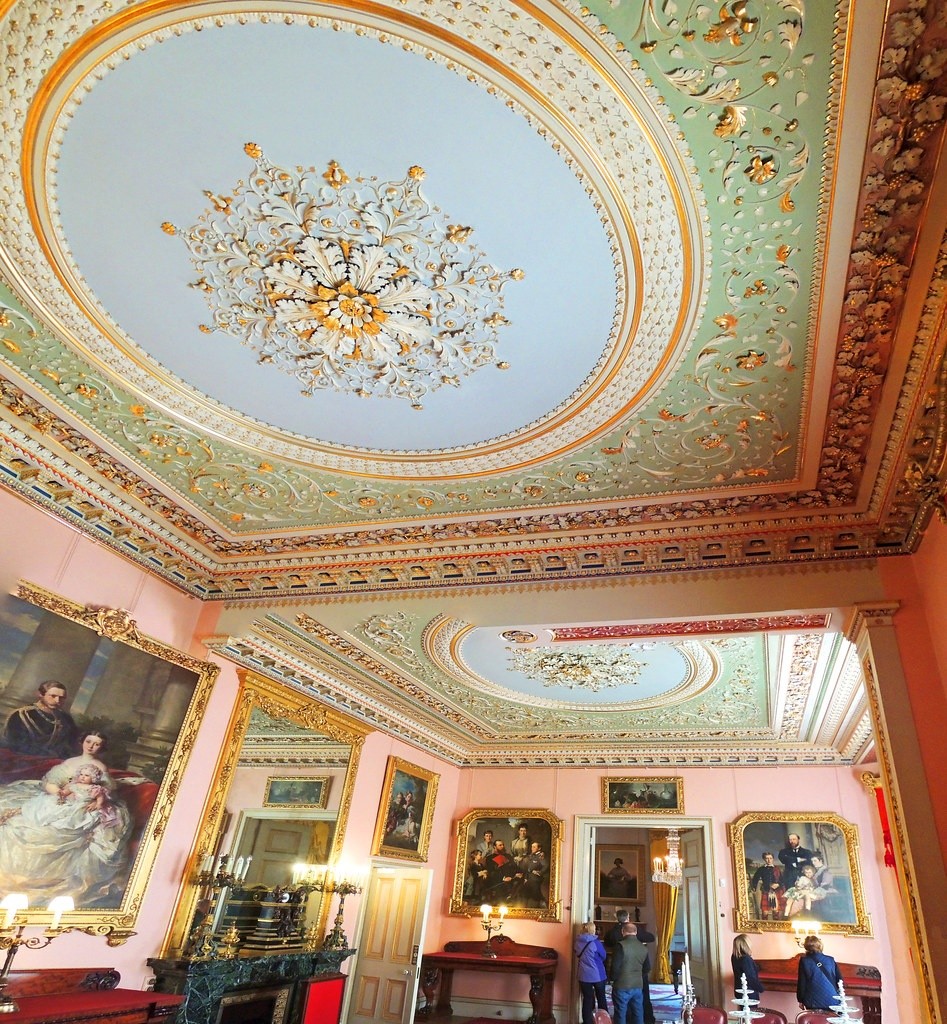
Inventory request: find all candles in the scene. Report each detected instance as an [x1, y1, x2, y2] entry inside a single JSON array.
[[197, 853, 253, 878], [686, 953, 691, 985], [681, 962, 686, 985]]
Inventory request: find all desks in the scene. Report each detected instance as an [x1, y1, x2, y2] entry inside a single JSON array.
[[0, 968, 185, 1024], [749, 953, 883, 1024], [415, 934, 559, 1024]]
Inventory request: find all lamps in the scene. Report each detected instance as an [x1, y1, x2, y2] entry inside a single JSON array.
[[653, 830, 683, 886], [477, 904, 510, 958]]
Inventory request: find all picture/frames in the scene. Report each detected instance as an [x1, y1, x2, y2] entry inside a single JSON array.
[[597, 843, 646, 908], [443, 806, 567, 924], [726, 810, 872, 938], [264, 776, 332, 810], [0, 583, 226, 950], [372, 755, 441, 865], [600, 776, 685, 815]]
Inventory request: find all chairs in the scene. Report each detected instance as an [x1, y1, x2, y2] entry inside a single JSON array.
[[795, 1010, 840, 1024], [683, 1003, 728, 1024], [746, 1008, 789, 1024]]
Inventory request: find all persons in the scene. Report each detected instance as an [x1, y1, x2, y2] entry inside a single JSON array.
[[731, 934, 765, 1024], [797, 936, 844, 1018], [605, 909, 656, 1024], [574, 922, 613, 1024]]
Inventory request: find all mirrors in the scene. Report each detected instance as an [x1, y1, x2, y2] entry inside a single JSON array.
[[166, 666, 379, 966]]
